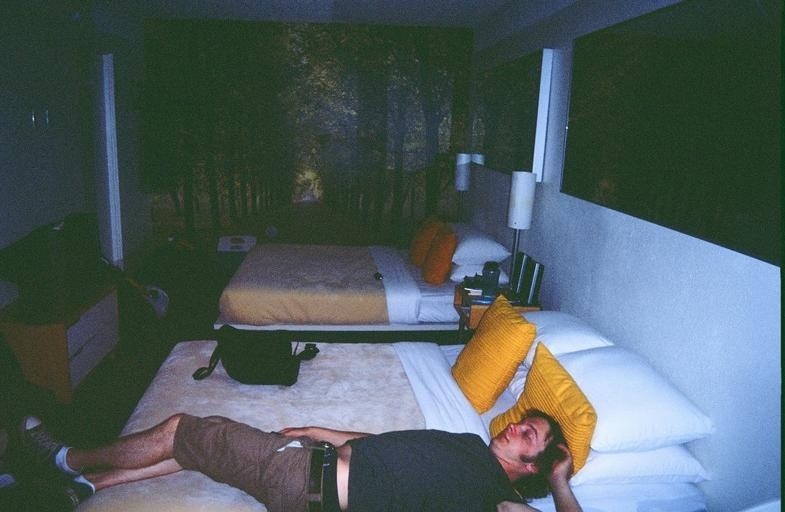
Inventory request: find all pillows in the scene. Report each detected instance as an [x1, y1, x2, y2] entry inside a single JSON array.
[[407, 214, 512, 286]]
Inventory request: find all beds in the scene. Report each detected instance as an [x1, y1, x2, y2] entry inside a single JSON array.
[[213, 242, 468, 332], [73, 339, 707, 512]]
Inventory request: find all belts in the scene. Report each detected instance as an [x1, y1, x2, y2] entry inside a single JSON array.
[[308, 440, 326, 510]]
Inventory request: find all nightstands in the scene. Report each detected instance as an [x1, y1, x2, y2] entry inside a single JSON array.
[[454, 286, 540, 344]]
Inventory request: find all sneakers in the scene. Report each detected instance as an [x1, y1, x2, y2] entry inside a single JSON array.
[[17, 416, 65, 476], [49, 479, 93, 510]]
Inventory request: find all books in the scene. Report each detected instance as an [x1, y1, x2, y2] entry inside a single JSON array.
[[461, 275, 494, 304]]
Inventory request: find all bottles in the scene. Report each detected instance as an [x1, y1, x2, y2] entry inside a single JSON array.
[[482, 261, 500, 300]]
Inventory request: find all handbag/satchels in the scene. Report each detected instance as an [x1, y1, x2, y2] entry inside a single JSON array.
[[214, 323, 299, 386]]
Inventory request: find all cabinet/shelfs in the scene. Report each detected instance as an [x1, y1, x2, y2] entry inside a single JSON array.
[[0, 279, 122, 406]]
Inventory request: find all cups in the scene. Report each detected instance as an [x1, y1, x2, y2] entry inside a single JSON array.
[[463, 277, 480, 289]]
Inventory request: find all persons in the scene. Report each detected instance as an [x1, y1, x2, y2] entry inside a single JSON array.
[[11, 407, 583, 511]]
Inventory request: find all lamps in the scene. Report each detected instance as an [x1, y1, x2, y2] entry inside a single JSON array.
[[455, 152, 471, 224], [499, 171, 537, 294]]
